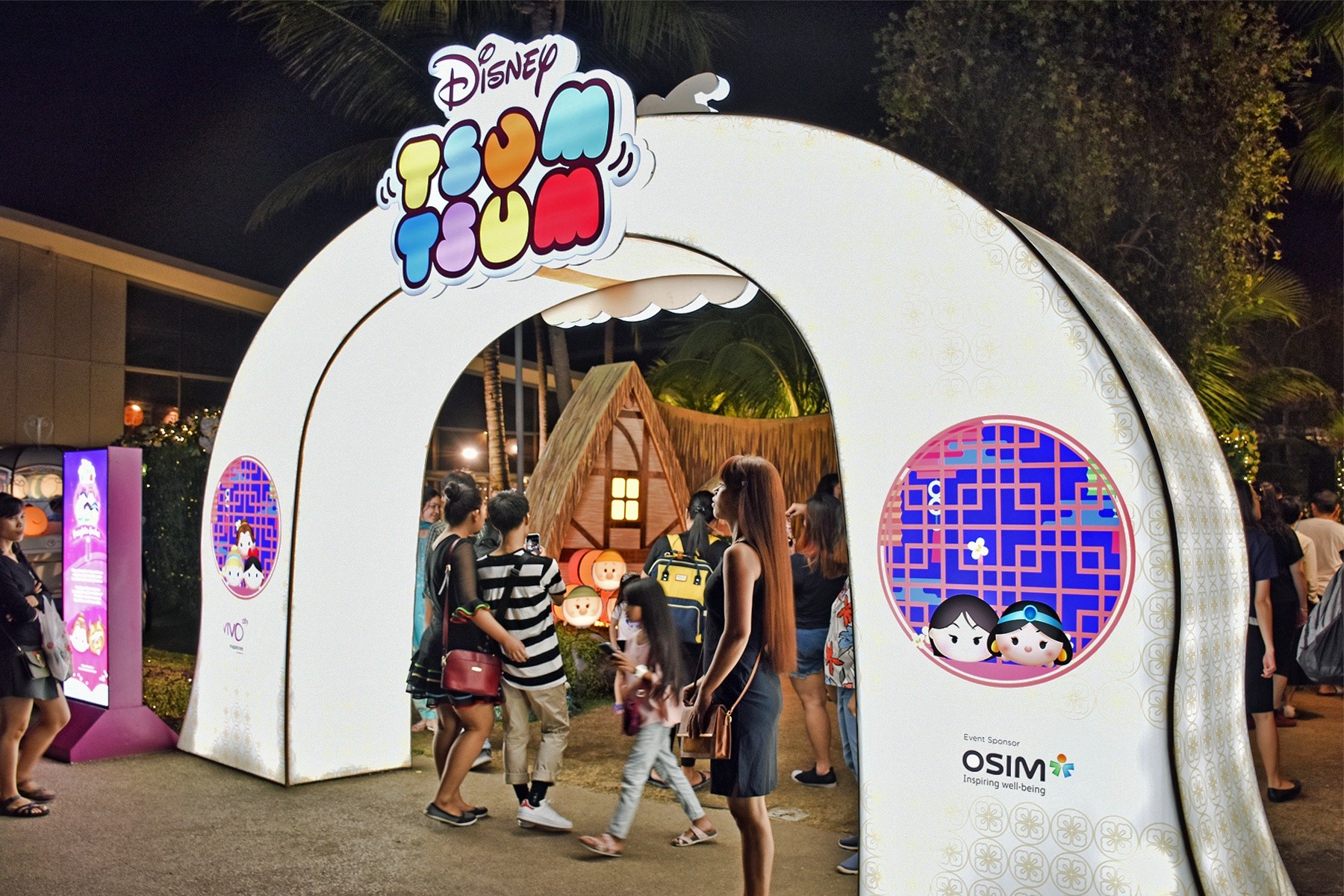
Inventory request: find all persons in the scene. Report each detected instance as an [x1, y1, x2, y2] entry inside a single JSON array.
[[1233, 474, 1344, 803], [414, 447, 860, 895], [0, 491, 73, 818]]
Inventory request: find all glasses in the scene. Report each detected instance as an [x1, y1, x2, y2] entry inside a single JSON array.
[[1252, 495, 1262, 502]]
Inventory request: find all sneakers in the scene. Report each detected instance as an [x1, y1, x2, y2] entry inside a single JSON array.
[[515, 797, 573, 829]]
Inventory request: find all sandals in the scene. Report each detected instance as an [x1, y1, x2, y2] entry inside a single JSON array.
[[2, 777, 56, 816]]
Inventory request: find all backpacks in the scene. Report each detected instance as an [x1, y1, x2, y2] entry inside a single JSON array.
[[646, 533, 723, 650]]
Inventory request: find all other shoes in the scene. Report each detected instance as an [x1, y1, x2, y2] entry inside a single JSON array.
[[1274, 708, 1296, 727], [467, 745, 493, 772], [424, 719, 437, 732], [838, 836, 859, 850], [836, 852, 859, 874], [441, 806, 489, 823], [790, 762, 838, 788], [1245, 712, 1255, 729], [423, 802, 477, 826], [1267, 778, 1301, 802], [411, 720, 426, 733]]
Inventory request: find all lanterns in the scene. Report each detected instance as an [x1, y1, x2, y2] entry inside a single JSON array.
[[125, 404, 143, 427]]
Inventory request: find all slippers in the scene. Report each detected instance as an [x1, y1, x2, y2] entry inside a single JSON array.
[[691, 770, 711, 790], [1317, 684, 1343, 695], [671, 823, 718, 847], [577, 832, 623, 858], [647, 766, 668, 789]]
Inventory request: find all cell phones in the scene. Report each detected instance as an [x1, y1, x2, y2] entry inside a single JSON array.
[[525, 533, 540, 556], [598, 643, 622, 662]]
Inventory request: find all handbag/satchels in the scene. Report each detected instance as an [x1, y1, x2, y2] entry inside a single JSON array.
[[622, 697, 644, 736], [37, 593, 74, 682], [441, 649, 500, 696], [23, 650, 48, 681], [676, 704, 731, 760]]
